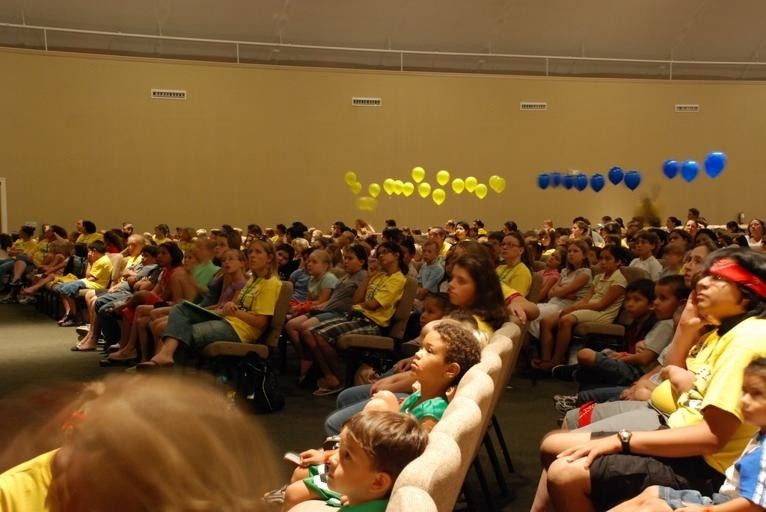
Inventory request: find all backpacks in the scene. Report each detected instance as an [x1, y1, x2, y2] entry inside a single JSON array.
[[237, 350, 285, 415]]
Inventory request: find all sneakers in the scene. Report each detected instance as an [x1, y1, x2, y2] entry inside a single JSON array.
[[551, 363, 579, 380], [1, 280, 36, 306], [552, 391, 580, 417]]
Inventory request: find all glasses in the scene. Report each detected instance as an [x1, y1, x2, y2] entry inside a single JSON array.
[[499, 242, 520, 248], [705, 273, 752, 295]]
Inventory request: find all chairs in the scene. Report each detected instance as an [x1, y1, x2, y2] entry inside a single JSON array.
[[0, 231, 766, 511]]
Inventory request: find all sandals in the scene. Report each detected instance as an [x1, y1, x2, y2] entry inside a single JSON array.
[[311, 379, 345, 398], [57, 313, 175, 376]]
